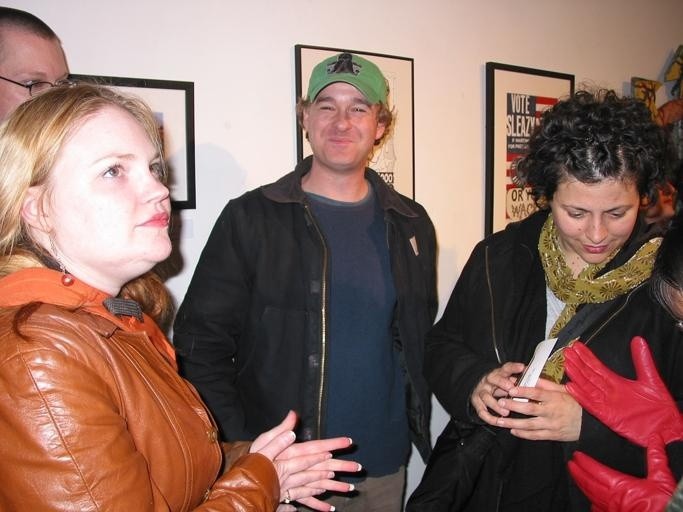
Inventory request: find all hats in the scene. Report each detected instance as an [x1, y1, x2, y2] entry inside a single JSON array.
[[307, 52, 387, 106]]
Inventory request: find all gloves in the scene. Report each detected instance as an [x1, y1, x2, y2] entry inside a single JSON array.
[[566, 432, 678, 512], [560, 336, 682, 450]]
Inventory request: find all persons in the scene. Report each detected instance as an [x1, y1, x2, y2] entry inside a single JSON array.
[[0, 86, 367, 511], [0, 4, 69, 134], [406, 90, 682, 512], [171, 50, 439, 509], [563, 205, 683, 512]]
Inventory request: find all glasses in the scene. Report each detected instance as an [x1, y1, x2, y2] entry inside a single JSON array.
[[0, 76, 78, 101]]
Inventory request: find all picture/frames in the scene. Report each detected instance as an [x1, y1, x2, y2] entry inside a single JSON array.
[[485, 65, 574, 240], [295, 46, 411, 208], [56, 74, 196, 209]]
